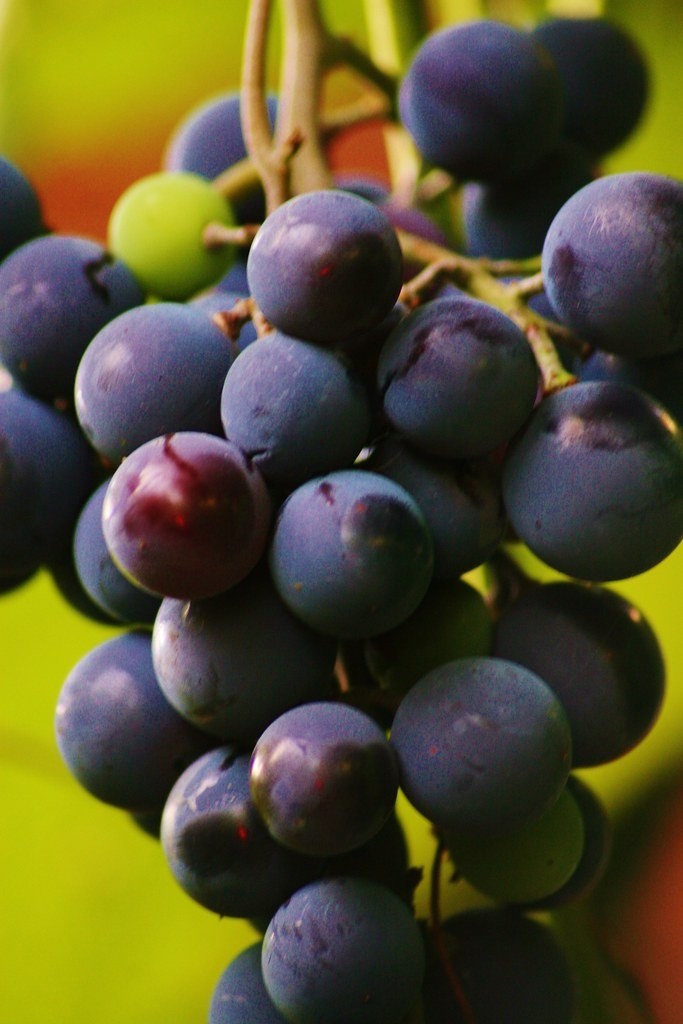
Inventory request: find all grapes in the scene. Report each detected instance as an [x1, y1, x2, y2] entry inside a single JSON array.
[[0, 16, 683, 1024]]
